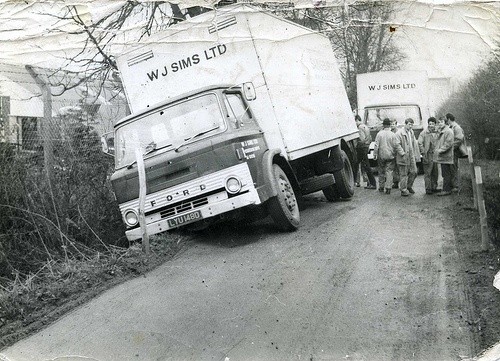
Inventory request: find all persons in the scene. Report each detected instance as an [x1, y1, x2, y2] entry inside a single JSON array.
[[418, 113, 464, 196], [390, 120, 401, 188], [391, 118, 421, 196], [354, 115, 376, 189], [373, 118, 405, 194]]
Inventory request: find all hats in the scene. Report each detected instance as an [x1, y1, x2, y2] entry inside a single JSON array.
[[382, 118, 390, 125]]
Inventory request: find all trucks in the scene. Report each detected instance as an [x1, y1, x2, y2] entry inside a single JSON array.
[[103, 2, 362, 241], [355, 70, 435, 168]]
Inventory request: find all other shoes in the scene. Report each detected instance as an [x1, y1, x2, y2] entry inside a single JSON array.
[[392, 182, 400, 189], [408, 187, 417, 194], [386, 190, 391, 194], [378, 187, 384, 192], [433, 186, 442, 192], [426, 188, 433, 194], [452, 186, 460, 192], [401, 191, 409, 196], [364, 184, 376, 189], [437, 188, 451, 196]]
